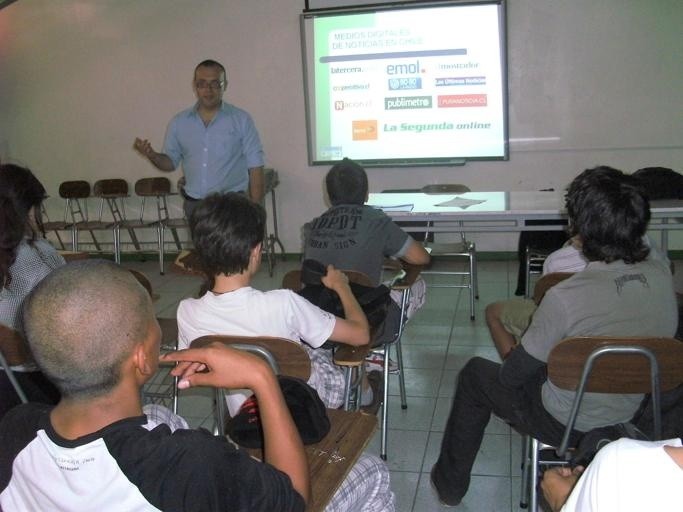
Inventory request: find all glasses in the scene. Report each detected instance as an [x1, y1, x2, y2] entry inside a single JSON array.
[[195, 80, 226, 89]]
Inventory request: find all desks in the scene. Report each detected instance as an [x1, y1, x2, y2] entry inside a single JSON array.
[[332, 310, 388, 366], [238, 408, 379, 512], [383, 258, 422, 290], [364, 190, 683, 257]]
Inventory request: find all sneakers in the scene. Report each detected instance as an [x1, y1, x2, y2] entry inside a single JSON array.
[[430, 463, 461, 507], [366, 352, 399, 375], [358, 370, 383, 416]]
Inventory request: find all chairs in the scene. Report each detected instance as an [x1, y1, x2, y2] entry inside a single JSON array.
[[524, 191, 572, 299], [337, 271, 407, 460], [262, 168, 287, 278], [42, 181, 98, 253], [520, 337, 683, 512], [405, 184, 479, 321], [1, 327, 34, 406], [161, 176, 194, 275], [117, 177, 180, 272], [75, 179, 139, 265]]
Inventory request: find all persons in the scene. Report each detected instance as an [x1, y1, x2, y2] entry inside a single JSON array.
[[486, 238, 587, 356], [539, 433, 682, 512], [429, 166, 678, 508], [0, 161, 66, 334], [302, 160, 427, 344], [175, 192, 383, 418], [132, 60, 265, 227], [2, 259, 396, 512]]
